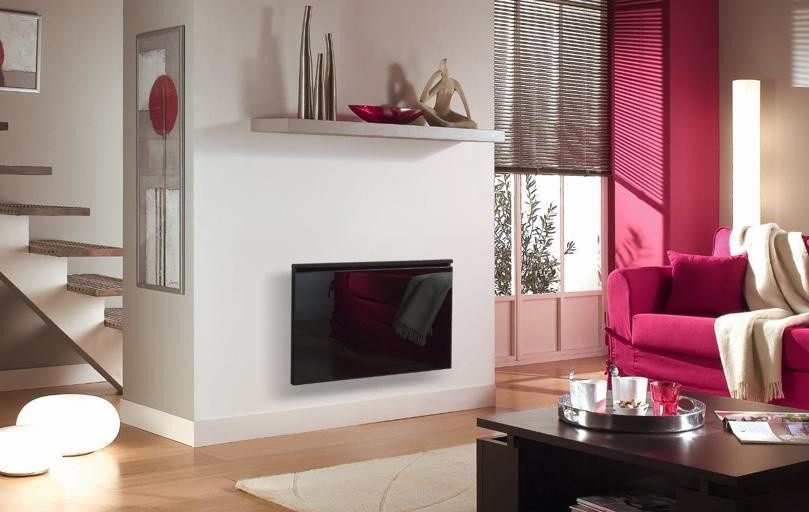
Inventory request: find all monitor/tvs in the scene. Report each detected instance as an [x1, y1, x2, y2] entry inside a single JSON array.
[[290, 258, 453, 386]]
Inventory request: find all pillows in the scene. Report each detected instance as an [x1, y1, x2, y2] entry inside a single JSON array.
[[660, 248, 748, 319]]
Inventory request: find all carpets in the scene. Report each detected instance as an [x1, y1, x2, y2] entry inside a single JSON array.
[[235, 440, 477, 512]]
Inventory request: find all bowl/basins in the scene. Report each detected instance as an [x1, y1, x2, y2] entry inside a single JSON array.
[[348, 105, 425, 125]]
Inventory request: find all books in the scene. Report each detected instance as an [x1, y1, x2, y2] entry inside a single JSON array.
[[568, 490, 677, 512], [713, 410, 809, 445]]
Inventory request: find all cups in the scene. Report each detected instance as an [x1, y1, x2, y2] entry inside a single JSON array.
[[651, 381, 681, 417], [568, 369, 607, 414], [609, 363, 648, 411]]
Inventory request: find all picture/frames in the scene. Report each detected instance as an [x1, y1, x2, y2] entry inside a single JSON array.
[[133, 23, 187, 295], [0, 10, 44, 94]]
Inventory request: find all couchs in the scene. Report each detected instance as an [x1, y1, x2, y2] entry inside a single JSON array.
[[603, 224, 809, 413]]
